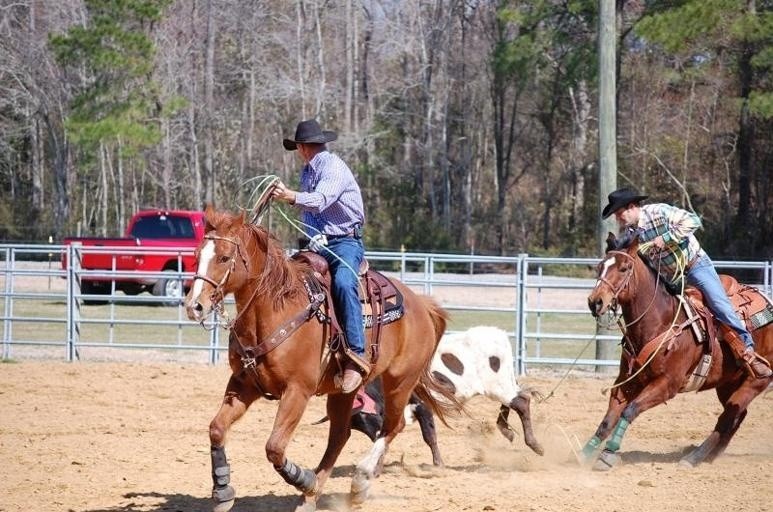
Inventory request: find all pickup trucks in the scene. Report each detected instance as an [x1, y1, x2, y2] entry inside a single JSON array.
[[61, 211, 209, 307]]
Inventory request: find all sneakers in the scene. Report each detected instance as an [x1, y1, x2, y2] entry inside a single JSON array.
[[743, 351, 772, 380]]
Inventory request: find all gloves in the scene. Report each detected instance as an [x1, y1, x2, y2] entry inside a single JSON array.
[[308, 232, 329, 253]]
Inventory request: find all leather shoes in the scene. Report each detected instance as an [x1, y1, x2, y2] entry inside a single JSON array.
[[339, 355, 369, 396]]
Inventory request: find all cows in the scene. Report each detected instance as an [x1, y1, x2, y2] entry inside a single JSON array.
[[311, 326, 545, 478]]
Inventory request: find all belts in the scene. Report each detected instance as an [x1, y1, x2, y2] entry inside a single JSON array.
[[298, 233, 348, 249], [683, 253, 700, 276]]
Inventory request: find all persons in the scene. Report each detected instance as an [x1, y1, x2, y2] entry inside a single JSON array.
[[271, 120, 366, 394], [602, 188, 773, 379]]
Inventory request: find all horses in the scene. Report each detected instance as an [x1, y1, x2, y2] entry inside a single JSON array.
[[184, 200, 479, 512], [574, 232, 773, 471]]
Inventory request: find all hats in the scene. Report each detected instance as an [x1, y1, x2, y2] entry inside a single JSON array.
[[281, 118, 339, 151], [600, 187, 649, 221]]
